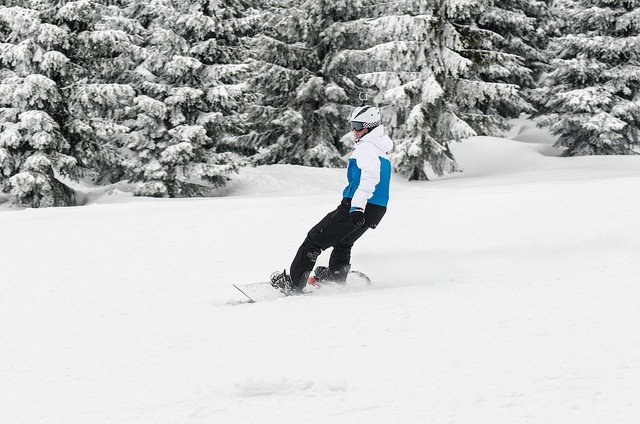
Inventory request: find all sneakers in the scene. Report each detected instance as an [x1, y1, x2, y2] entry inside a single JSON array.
[[270, 270, 296, 296], [314, 266, 330, 284]]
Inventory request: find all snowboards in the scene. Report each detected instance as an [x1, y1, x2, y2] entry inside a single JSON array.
[[233, 271, 372, 303]]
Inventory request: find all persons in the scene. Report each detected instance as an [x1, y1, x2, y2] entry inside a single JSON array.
[[268, 104, 395, 296]]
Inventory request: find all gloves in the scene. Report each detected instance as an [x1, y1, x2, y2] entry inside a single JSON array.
[[348, 211, 366, 228]]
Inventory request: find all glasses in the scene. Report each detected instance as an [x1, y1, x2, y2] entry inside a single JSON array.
[[351, 121, 363, 131]]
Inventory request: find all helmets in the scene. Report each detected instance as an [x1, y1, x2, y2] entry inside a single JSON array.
[[350, 105, 380, 128]]
